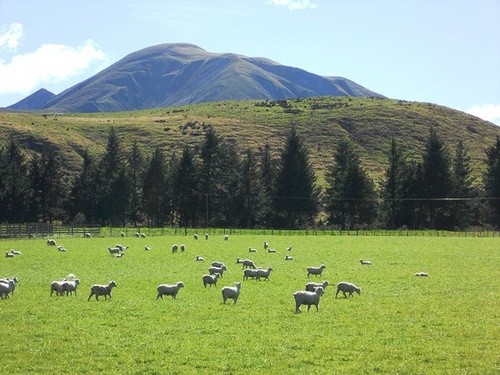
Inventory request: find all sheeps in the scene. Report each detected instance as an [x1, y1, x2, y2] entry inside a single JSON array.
[[263, 242, 269, 250], [203, 261, 227, 288], [221, 282, 241, 305], [4, 232, 151, 259], [224, 235, 230, 240], [268, 248, 276, 253], [172, 245, 178, 253], [360, 259, 373, 264], [195, 256, 204, 261], [306, 264, 327, 276], [248, 248, 257, 252], [236, 257, 273, 282], [0, 273, 81, 299], [286, 247, 292, 251], [87, 280, 117, 302], [293, 287, 325, 313], [193, 234, 199, 240], [413, 272, 428, 277], [180, 244, 185, 252], [285, 256, 293, 259], [335, 282, 361, 297], [306, 281, 329, 295], [205, 234, 209, 239], [156, 282, 184, 300]]
